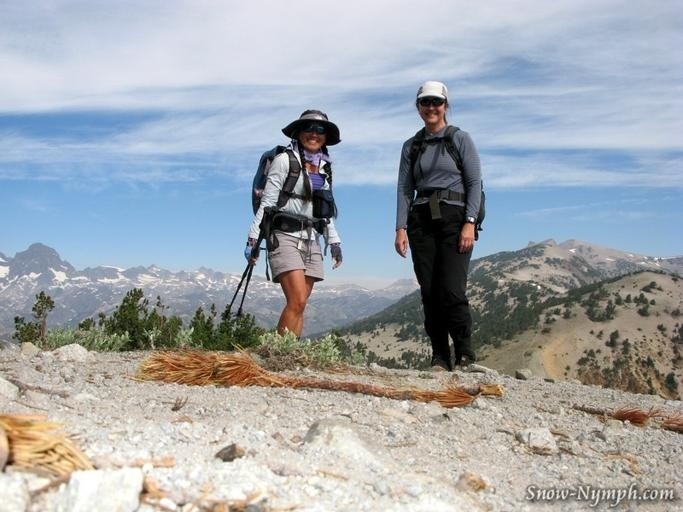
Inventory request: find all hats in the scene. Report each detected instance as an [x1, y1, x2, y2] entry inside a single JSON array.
[[282, 110, 341, 145], [417, 81, 448, 100]]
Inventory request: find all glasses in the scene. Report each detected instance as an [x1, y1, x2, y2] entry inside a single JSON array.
[[305, 125, 324, 135], [419, 97, 444, 107]]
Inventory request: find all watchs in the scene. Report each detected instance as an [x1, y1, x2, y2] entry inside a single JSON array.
[[465, 216, 477, 224]]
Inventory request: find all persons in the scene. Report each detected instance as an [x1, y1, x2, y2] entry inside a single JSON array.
[[244, 108, 343, 343], [393, 80, 483, 374]]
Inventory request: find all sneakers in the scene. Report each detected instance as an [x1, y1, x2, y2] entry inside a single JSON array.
[[431, 355, 451, 371], [454, 356, 474, 370]]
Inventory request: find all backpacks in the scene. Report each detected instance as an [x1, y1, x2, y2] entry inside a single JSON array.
[[252, 145, 325, 235], [410, 127, 485, 241]]
